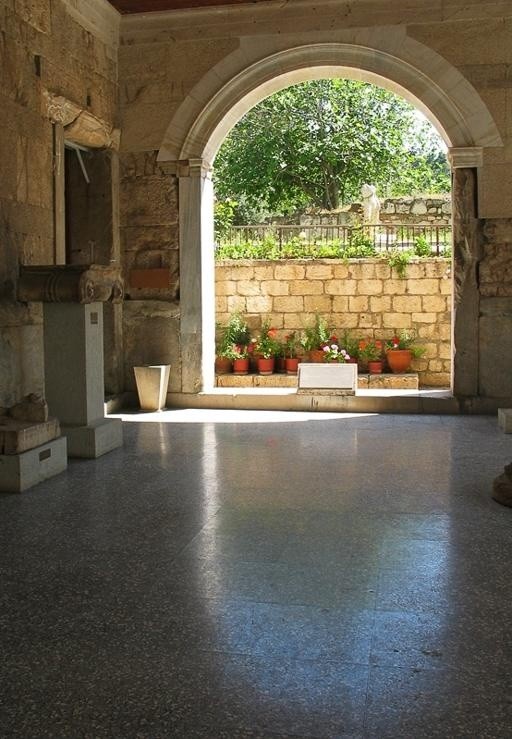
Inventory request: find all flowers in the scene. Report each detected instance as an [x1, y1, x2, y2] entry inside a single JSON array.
[[216, 315, 425, 364]]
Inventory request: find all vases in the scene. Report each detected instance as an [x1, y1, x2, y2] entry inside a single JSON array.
[[215, 350, 415, 392]]
